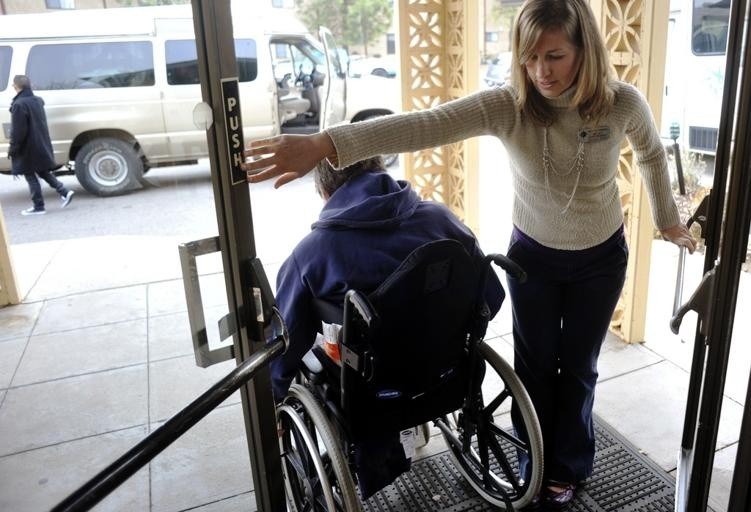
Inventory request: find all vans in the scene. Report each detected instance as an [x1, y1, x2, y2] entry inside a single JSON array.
[[0, 4, 400, 198]]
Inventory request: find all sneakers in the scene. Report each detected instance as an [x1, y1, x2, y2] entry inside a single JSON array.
[[61, 190, 74, 207], [22, 206, 45, 215]]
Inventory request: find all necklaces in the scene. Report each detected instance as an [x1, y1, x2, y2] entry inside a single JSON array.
[[542, 117, 584, 215]]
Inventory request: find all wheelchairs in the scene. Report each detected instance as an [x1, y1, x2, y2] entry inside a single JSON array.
[[274, 254, 544, 511]]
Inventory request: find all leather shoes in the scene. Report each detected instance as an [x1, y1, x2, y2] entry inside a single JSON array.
[[545, 485, 574, 507]]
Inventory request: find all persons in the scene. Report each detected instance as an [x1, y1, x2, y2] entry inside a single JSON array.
[[241, 0, 697, 508], [266, 159, 506, 428], [8, 75, 75, 215]]
